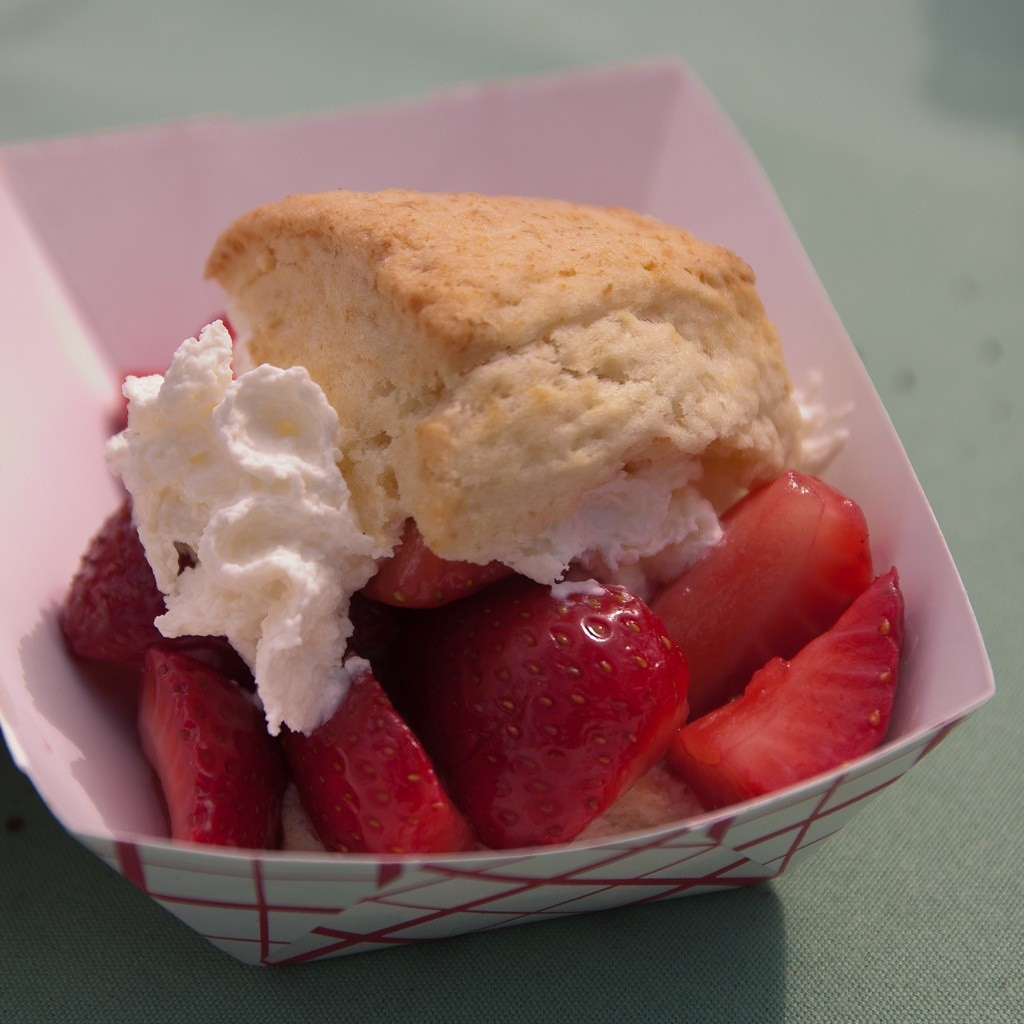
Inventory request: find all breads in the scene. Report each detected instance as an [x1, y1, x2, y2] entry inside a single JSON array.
[[203, 190, 838, 580]]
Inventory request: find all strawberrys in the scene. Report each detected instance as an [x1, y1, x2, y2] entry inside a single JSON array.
[[56, 313, 907, 859]]
